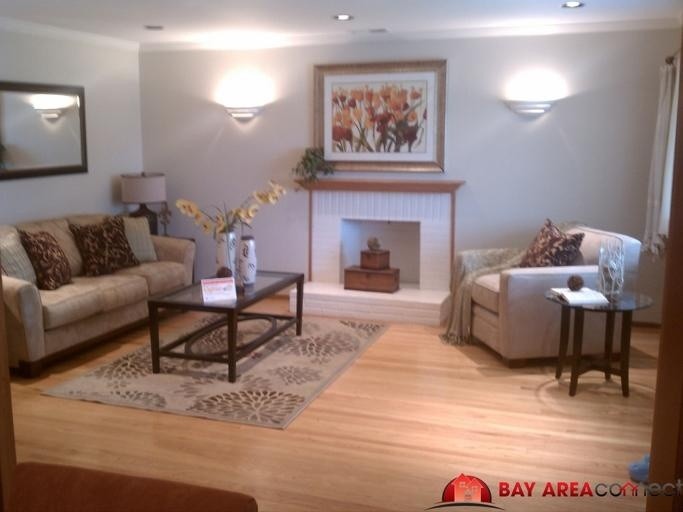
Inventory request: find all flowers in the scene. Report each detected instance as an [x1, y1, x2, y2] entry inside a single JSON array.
[[175, 178, 286, 234]]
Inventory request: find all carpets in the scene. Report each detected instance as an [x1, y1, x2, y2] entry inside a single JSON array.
[[38, 314, 388, 431]]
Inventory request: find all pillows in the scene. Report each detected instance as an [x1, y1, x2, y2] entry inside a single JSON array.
[[515, 218, 585, 268], [15, 214, 141, 291]]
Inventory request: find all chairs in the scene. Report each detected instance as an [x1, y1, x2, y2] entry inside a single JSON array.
[[0, 278, 257, 511], [450, 223, 643, 370]]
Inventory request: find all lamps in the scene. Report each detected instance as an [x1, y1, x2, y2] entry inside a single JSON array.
[[29, 93, 75, 120], [119, 170, 167, 235]]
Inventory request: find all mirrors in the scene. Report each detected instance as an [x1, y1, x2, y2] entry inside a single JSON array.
[[0, 81, 89, 179]]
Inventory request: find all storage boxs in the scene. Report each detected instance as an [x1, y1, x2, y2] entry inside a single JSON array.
[[340, 248, 399, 293]]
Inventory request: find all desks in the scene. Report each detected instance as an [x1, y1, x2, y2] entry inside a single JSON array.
[[542, 290, 653, 398]]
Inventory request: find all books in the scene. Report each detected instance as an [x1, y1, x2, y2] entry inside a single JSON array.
[[200, 276, 237, 305], [549, 282, 611, 308]]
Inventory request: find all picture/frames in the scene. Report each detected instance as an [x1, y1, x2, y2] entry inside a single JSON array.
[[311, 58, 448, 173]]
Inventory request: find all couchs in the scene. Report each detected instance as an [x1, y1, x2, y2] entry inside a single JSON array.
[[0, 213, 196, 380]]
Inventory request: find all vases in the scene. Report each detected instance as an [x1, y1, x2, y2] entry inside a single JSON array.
[[214, 235, 258, 286]]
